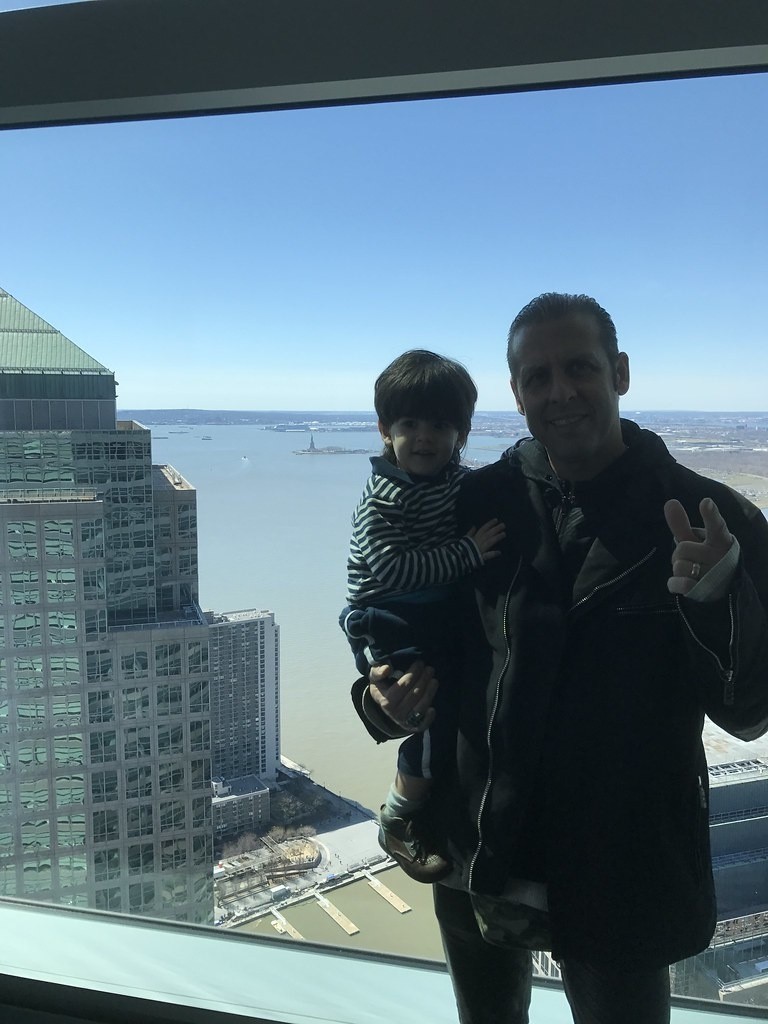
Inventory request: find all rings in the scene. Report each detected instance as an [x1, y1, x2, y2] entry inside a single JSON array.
[[692, 563, 700, 579], [405, 713, 423, 727]]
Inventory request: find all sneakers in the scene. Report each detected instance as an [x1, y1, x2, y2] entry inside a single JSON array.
[[377, 803, 452, 884]]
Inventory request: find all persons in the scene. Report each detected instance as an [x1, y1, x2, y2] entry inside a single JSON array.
[[339, 350, 505, 883], [350, 291, 768, 1024]]
